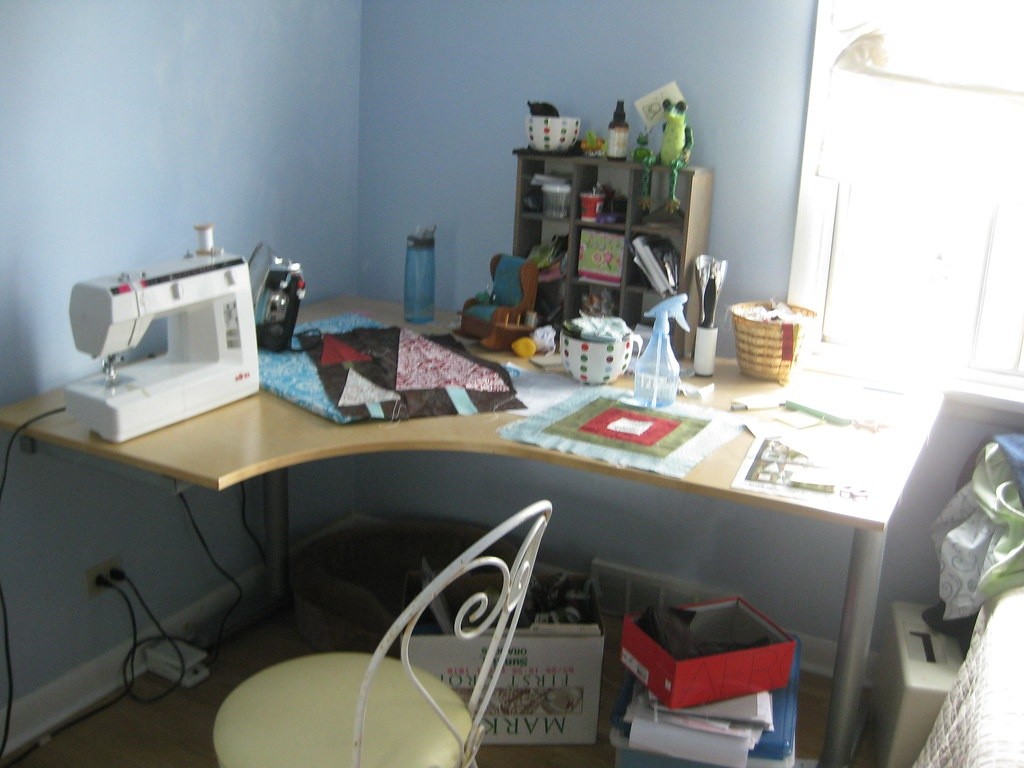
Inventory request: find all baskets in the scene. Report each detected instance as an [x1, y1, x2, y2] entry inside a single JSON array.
[[731, 302, 817, 383]]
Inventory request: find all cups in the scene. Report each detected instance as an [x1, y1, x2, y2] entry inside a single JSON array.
[[581, 194, 606, 221]]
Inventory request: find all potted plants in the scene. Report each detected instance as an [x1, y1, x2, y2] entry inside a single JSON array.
[[525, 99, 582, 150]]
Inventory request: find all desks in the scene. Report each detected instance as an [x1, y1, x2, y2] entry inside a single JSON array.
[[0, 291, 945, 768]]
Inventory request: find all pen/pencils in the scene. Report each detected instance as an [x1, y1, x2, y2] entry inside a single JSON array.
[[730, 402, 786, 411], [785, 400, 848, 426]]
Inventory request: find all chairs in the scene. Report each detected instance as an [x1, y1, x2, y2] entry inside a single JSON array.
[[452, 255, 539, 351], [212, 499, 554, 768]]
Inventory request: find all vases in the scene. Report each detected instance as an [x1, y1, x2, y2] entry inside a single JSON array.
[[558, 313, 633, 384]]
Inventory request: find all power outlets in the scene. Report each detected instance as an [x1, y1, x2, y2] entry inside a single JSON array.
[[84, 556, 126, 597]]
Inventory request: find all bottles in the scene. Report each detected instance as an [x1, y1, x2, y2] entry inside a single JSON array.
[[606, 100, 630, 162], [405, 225, 437, 322]]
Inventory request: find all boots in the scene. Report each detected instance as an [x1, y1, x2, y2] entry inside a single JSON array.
[[646, 604, 773, 664]]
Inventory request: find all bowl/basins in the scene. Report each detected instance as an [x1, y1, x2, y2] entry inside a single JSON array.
[[526, 117, 581, 151], [540, 185, 572, 221], [558, 331, 630, 385]]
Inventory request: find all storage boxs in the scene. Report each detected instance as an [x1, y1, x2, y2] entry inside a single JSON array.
[[577, 227, 626, 282], [878, 601, 964, 768], [609, 635, 803, 768], [395, 561, 603, 747], [619, 598, 797, 708]]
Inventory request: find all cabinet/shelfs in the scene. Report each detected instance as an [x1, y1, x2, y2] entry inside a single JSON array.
[[512, 148, 713, 359]]
